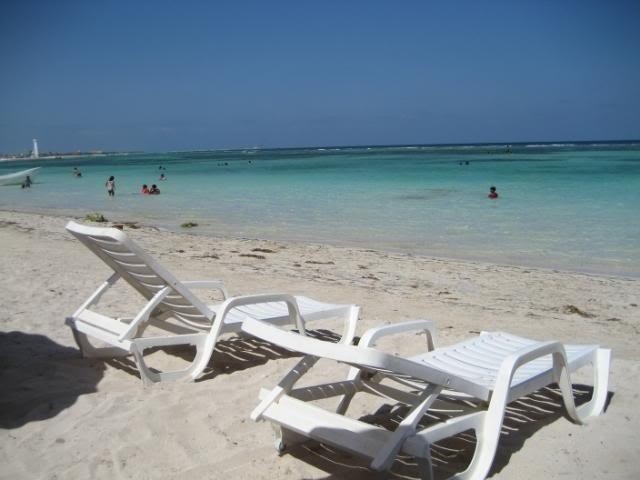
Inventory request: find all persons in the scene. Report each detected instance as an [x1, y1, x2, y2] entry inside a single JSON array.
[[141, 183, 162, 194], [106, 175, 116, 196], [488, 185, 498, 199], [74, 168, 82, 178], [22, 174, 33, 188], [158, 172, 167, 180]]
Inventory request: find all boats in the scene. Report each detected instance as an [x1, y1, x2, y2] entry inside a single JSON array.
[[0, 167, 44, 186]]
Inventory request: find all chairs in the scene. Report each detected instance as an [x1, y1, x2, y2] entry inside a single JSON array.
[[240, 304, 611, 480], [66, 221, 348, 387]]
